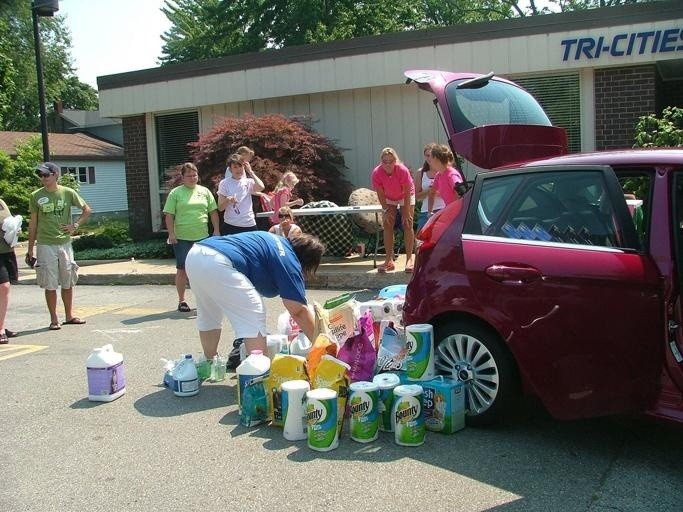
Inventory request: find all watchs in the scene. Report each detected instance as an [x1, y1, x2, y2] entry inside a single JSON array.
[[73, 222, 79, 230]]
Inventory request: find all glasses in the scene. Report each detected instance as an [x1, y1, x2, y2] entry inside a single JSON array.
[[38, 173, 54, 177], [233, 201, 240, 214], [279, 214, 290, 218]]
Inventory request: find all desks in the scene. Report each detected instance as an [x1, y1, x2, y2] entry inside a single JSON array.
[[255, 204, 399, 267]]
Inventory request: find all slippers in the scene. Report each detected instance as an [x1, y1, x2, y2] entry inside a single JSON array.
[[5, 329, 18, 337], [0, 333, 9, 344], [63, 316, 86, 324], [178, 302, 190, 312], [49, 322, 61, 330]]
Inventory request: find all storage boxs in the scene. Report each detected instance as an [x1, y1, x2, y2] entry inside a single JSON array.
[[392, 371, 466, 435]]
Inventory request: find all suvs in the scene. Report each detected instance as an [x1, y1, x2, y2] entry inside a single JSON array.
[[400, 68, 682, 428]]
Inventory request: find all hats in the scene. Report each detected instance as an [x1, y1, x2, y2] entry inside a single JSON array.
[[35, 162, 59, 174]]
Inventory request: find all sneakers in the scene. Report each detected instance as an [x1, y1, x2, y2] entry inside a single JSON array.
[[405, 264, 414, 273], [378, 261, 394, 273]]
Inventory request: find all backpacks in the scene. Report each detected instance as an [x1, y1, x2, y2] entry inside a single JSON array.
[[260, 186, 277, 225]]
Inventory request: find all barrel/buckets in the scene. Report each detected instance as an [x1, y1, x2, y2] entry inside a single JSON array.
[[290, 330, 313, 356], [86, 344, 126, 401], [236, 350, 270, 415]]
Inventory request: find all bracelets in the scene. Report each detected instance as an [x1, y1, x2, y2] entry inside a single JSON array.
[[381, 207, 389, 213]]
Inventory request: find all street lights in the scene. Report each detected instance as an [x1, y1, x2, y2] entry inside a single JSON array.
[[30, 0, 59, 162]]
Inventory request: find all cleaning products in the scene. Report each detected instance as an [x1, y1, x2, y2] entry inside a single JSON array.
[[211, 356, 219, 380], [199, 351, 211, 379], [215, 358, 226, 381]]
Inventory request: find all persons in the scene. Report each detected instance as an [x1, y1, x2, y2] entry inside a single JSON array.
[[26, 162, 90, 329], [163, 163, 220, 312], [269, 172, 304, 226], [372, 147, 415, 273], [216, 154, 265, 236], [185, 230, 325, 370], [415, 143, 445, 235], [268, 206, 302, 240], [428, 143, 464, 220], [224, 146, 254, 180], [0, 198, 23, 344]]
